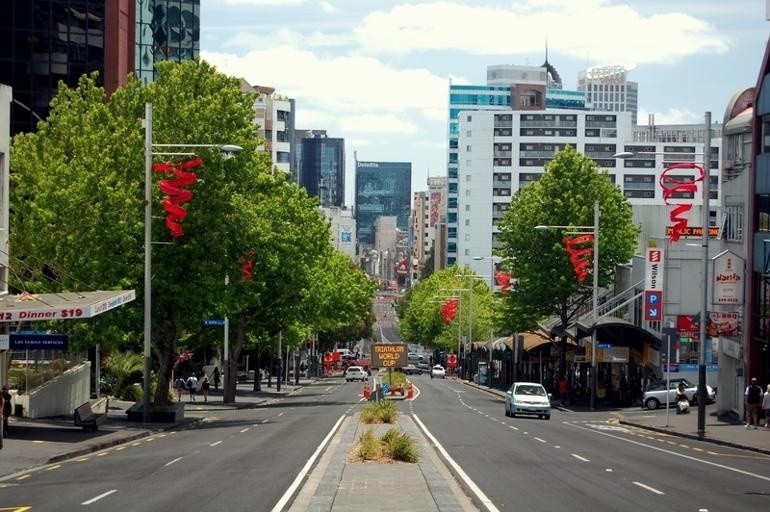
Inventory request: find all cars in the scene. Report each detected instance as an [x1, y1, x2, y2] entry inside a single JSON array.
[[430, 363, 446, 379], [505, 382, 553, 420], [641, 378, 716, 410], [394, 363, 424, 375], [344, 365, 369, 382]]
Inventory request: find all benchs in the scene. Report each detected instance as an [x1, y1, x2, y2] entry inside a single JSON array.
[[73, 402, 108, 430]]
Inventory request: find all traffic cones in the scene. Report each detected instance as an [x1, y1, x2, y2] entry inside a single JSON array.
[[406, 382, 414, 399], [363, 381, 371, 400]]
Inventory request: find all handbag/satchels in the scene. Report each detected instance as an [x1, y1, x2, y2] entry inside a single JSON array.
[[187, 380, 192, 387]]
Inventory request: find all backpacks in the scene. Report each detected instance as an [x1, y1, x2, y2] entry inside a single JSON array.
[[177, 381, 182, 387]]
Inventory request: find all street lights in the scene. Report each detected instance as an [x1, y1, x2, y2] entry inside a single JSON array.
[[428, 256, 495, 390], [533, 199, 600, 414], [140, 101, 245, 424], [471, 251, 520, 382], [612, 111, 713, 436]]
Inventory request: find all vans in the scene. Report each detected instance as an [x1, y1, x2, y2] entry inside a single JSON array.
[[333, 348, 356, 363]]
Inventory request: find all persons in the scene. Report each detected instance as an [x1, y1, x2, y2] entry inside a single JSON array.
[[185, 372, 198, 402], [559, 376, 569, 407], [760, 384, 770, 428], [0, 385, 13, 439], [210, 366, 221, 389], [743, 378, 764, 429], [202, 377, 209, 401], [675, 383, 686, 414], [175, 373, 185, 401]]
